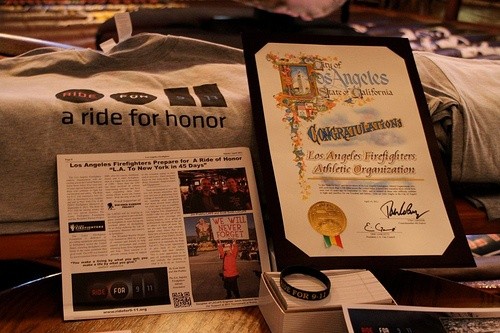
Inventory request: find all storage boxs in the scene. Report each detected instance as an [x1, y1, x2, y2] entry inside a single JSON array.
[[259, 267, 396, 332]]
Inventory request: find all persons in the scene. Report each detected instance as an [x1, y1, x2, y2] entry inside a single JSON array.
[[178, 167, 253, 213], [215, 231, 238, 285], [217, 243, 241, 298]]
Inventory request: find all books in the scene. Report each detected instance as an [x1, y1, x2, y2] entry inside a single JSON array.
[[54, 146, 274, 324]]
